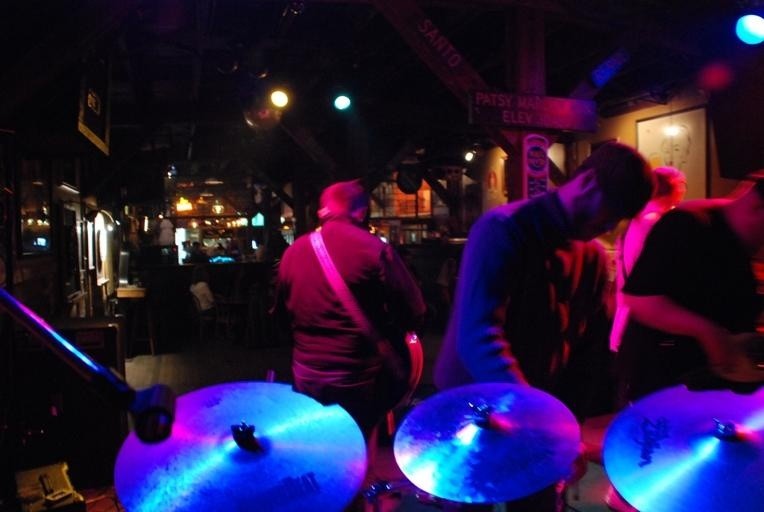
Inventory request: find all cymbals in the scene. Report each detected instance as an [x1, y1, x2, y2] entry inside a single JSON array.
[[394, 383, 580, 504], [116, 382, 369, 510], [603, 383, 764, 512]]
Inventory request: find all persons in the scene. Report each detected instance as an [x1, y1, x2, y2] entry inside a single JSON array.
[[117, 227, 292, 348], [431, 140, 653, 511], [422, 220, 454, 336], [277, 180, 428, 447], [615, 175, 764, 410], [606, 166, 686, 409]]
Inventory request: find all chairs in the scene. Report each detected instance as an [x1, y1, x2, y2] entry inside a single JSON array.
[[192, 296, 220, 338]]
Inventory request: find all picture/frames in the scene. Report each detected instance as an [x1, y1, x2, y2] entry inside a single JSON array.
[[635, 103, 711, 199], [1, 226, 13, 298]]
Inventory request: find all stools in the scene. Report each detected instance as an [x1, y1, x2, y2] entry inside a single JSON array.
[[129, 295, 157, 356]]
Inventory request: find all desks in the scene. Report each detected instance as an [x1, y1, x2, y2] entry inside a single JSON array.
[[221, 296, 248, 344]]
